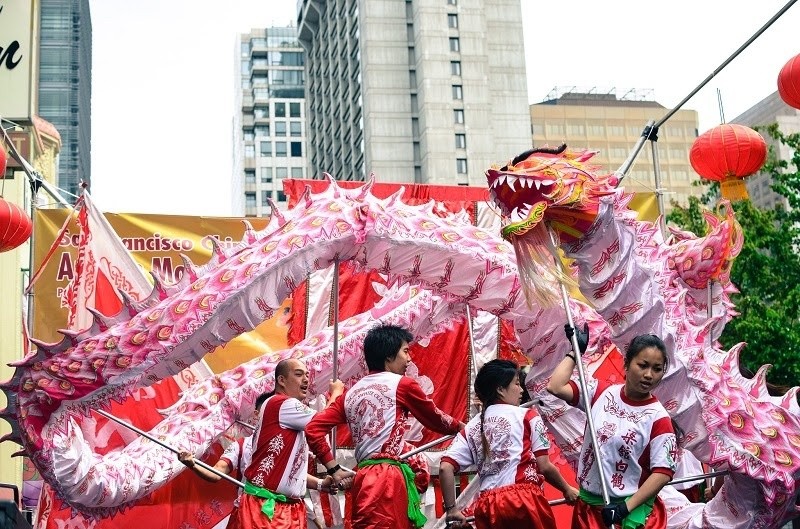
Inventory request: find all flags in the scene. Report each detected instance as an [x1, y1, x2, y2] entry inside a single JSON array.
[[22, 294, 31, 358]]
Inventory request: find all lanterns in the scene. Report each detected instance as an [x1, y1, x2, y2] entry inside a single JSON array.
[[777, 53, 800, 110], [689, 88, 767, 201], [0, 144, 6, 177], [0, 196, 33, 253]]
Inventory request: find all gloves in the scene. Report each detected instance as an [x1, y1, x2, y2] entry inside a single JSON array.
[[602, 501, 630, 525], [564, 322, 589, 356]]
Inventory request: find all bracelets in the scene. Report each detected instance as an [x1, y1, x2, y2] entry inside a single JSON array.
[[317, 479, 324, 491], [443, 504, 455, 511], [327, 464, 341, 474], [566, 354, 576, 363], [711, 486, 716, 495]]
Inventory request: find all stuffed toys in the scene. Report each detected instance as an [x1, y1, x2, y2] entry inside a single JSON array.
[[1, 144, 800, 529]]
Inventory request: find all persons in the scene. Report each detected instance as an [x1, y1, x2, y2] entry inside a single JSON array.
[[439, 359, 579, 529], [178, 392, 338, 529], [225, 359, 345, 529], [671, 418, 724, 503], [304, 325, 466, 529], [548, 322, 677, 529]]
